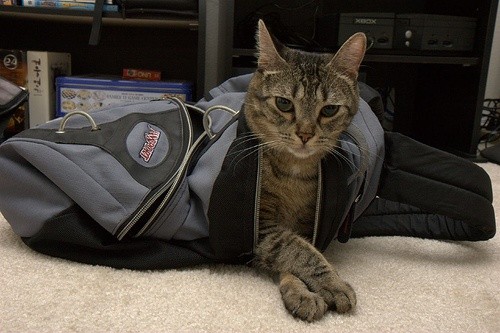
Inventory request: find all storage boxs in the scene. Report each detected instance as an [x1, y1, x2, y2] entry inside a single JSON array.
[[55, 72, 191, 117]]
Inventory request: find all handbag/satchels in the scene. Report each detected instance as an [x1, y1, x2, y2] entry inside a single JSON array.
[[0, 73, 496, 270]]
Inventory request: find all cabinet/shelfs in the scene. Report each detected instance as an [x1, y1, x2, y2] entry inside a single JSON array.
[[205, 1, 497, 161]]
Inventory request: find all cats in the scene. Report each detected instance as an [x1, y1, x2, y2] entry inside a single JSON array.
[[244, 18, 368, 323]]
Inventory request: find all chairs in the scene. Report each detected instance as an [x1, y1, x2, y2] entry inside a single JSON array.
[[476, 98, 500, 165]]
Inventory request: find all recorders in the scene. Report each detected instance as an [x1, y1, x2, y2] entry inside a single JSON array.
[[336, 9, 476, 55]]
